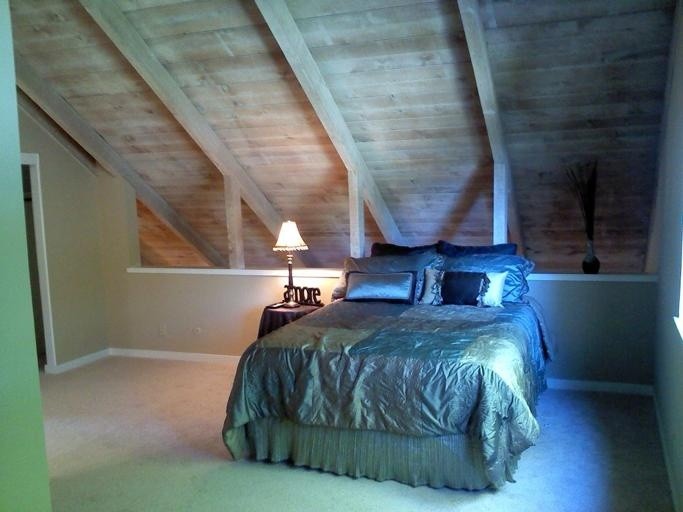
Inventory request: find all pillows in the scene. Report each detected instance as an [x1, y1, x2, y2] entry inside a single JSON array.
[[419, 268, 509, 308], [344, 270, 417, 306], [443, 255, 536, 302], [439, 239, 517, 257], [371, 242, 431, 256], [332, 249, 447, 307]]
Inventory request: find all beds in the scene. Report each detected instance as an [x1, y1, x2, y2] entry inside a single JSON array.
[[222, 298, 553, 491]]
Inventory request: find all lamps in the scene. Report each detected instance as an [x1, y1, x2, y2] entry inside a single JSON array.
[[272, 220, 309, 306]]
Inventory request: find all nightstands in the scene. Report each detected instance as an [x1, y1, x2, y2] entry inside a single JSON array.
[[257, 302, 325, 337]]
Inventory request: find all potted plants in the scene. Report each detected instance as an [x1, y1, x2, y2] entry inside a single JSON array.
[[565, 158, 600, 273]]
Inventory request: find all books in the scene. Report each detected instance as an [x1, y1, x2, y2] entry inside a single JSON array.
[[264, 302, 284, 309]]
